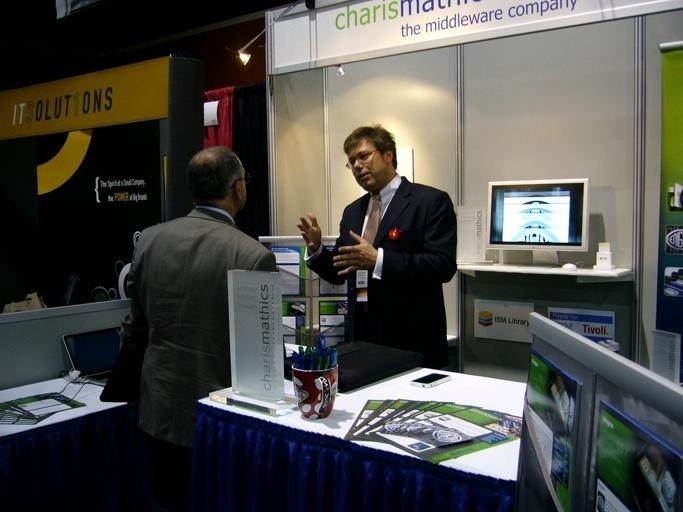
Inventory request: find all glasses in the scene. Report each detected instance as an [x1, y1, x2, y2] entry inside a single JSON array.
[[346, 150, 376, 169], [230, 171, 252, 190]]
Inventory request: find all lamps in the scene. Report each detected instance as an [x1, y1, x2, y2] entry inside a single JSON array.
[[237, 0, 315, 68]]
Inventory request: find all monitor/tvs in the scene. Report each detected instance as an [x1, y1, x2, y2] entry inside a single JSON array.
[[486, 178, 590, 269]]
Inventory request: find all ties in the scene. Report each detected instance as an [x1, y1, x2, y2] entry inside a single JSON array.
[[362, 193, 381, 247]]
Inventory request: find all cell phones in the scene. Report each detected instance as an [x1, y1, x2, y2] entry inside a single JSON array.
[[411, 373, 452, 387]]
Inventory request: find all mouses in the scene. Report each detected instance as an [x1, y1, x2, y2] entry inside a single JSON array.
[[562, 263, 577, 271]]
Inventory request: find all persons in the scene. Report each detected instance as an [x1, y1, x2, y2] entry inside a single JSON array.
[[120, 145, 277, 512], [297, 124, 457, 369]]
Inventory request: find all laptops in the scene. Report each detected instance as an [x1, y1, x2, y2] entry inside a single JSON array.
[[61, 326, 122, 387]]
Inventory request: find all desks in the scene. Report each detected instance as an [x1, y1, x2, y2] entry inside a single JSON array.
[[194, 364, 527, 511], [2, 371, 135, 510]]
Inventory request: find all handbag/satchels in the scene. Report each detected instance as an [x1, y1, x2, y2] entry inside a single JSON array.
[[284, 338, 423, 395]]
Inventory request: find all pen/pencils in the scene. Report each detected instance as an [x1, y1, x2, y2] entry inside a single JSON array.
[[292, 336, 337, 370]]
